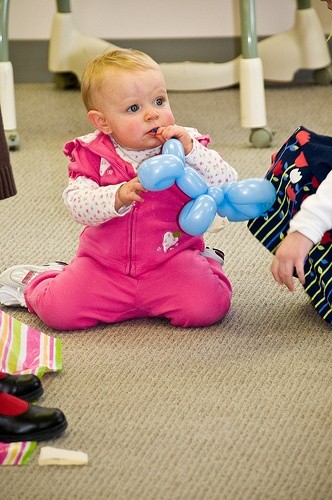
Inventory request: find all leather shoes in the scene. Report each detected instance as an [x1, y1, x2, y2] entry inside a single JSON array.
[[0, 373, 44, 404], [0, 401, 67, 444]]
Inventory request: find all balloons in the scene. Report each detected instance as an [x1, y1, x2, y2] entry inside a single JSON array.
[[138, 139, 275, 237]]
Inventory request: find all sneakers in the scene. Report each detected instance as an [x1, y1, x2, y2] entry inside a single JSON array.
[[200, 247, 224, 265], [0, 260, 68, 307]]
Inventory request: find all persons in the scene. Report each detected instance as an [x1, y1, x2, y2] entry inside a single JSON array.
[[269, 168, 332, 290], [0, 109, 69, 441], [0, 49, 240, 332]]
